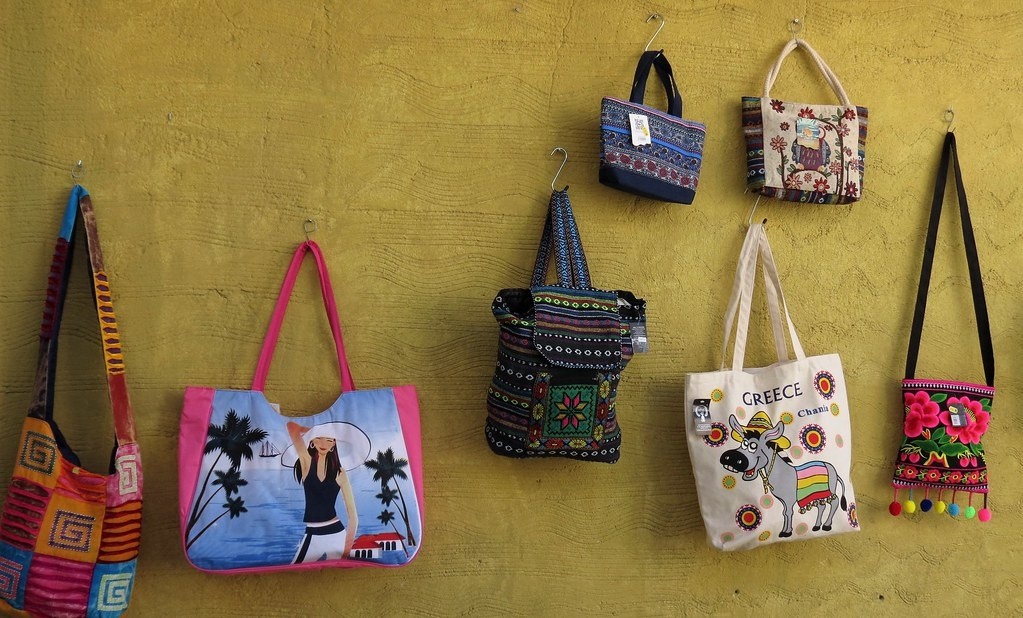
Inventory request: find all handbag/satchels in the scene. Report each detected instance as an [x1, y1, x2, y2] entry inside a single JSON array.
[[890, 133, 995, 523], [683, 222, 860, 552], [741, 38, 869, 205], [597, 52, 705, 205], [0, 185, 143, 618], [177, 246, 425, 574], [483, 189, 645, 465]]
